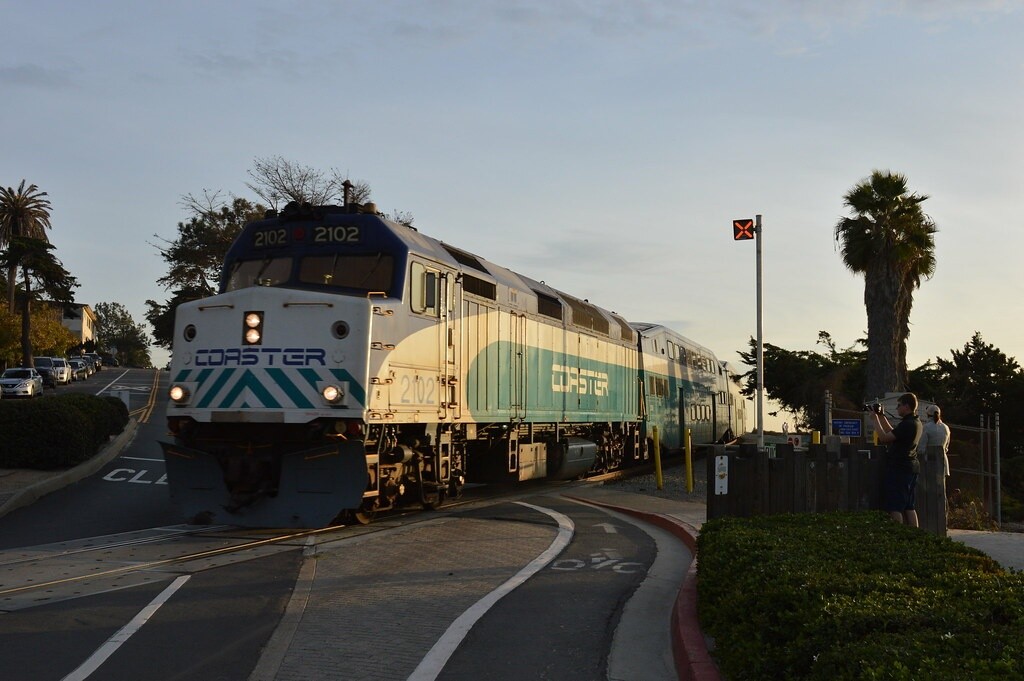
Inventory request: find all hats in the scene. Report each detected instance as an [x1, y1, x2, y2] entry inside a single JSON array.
[[926, 404, 940, 416]]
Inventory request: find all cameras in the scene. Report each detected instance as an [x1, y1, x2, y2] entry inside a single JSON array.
[[862, 403, 883, 415]]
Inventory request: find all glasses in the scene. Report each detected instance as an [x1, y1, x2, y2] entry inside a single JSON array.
[[898, 404, 904, 408]]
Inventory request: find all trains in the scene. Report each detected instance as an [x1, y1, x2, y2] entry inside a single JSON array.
[[166, 179, 745, 526]]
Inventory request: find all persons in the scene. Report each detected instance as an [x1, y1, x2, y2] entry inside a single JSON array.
[[915, 404, 950, 538], [863, 393, 923, 527]]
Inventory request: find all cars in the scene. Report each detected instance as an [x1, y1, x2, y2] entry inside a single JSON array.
[[72, 356, 96, 376], [0, 368, 43, 399], [67, 360, 88, 382], [34, 356, 57, 389], [85, 353, 102, 372], [52, 358, 71, 385]]
